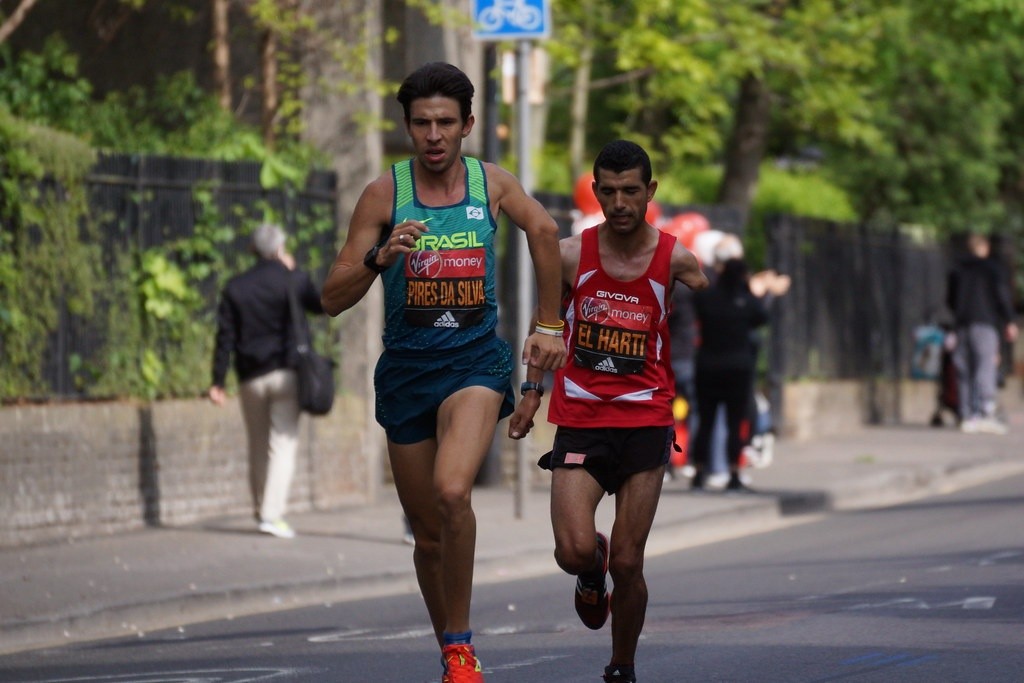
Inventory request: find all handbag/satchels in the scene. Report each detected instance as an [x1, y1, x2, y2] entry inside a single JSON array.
[[286, 272, 338, 415]]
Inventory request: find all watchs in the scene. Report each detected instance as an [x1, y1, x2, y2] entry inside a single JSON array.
[[364, 244, 390, 273]]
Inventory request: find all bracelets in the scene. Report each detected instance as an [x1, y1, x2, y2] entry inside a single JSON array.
[[521, 383, 544, 396], [536, 320, 564, 336]]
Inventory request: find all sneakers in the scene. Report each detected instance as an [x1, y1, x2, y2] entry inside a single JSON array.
[[599, 665, 636, 683], [575, 532, 611, 630], [440, 642, 484, 683]]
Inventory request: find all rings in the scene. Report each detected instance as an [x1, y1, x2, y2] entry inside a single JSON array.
[[400, 235, 403, 245]]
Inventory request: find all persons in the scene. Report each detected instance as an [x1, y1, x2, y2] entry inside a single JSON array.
[[931, 232, 1018, 434], [690, 235, 792, 492], [508, 141, 708, 683], [320, 62, 567, 683], [210, 227, 325, 538]]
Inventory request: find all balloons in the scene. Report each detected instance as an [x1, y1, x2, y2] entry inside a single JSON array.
[[571, 171, 724, 267]]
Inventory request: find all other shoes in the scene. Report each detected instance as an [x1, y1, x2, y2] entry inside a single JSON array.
[[963, 417, 1006, 434], [257, 519, 295, 539], [404, 534, 415, 546], [690, 472, 705, 486], [728, 480, 742, 488]]
